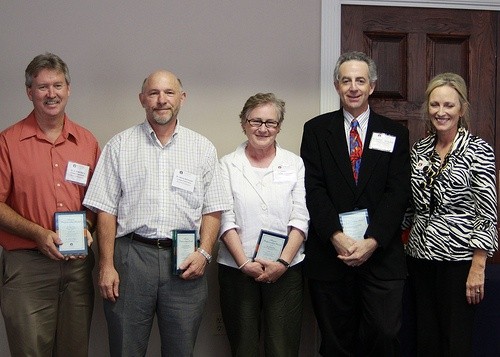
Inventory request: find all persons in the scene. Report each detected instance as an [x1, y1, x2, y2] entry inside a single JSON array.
[[300, 51, 411, 357], [0, 52, 101, 357], [403, 73, 498, 357], [82, 72, 230, 357], [218, 93, 310, 357]]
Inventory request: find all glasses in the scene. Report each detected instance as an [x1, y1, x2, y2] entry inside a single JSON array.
[[246, 118, 280, 128]]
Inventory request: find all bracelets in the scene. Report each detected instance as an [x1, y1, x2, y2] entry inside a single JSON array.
[[197, 248, 212, 265], [238, 259, 249, 269], [277, 258, 290, 269]]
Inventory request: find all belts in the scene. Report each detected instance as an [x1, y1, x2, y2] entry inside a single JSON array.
[[125, 232, 173, 248]]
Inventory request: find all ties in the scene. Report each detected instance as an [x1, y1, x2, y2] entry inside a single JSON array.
[[349, 119, 363, 187]]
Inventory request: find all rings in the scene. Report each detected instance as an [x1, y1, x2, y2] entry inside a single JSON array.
[[475, 292, 480, 294], [267, 282, 271, 283]]
